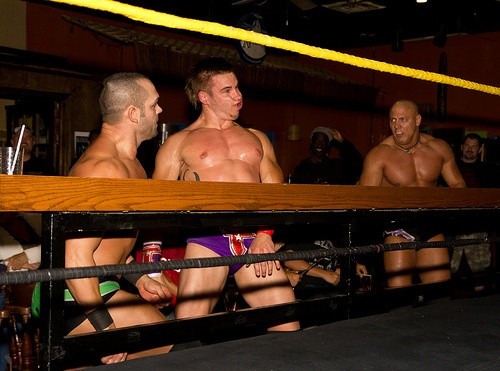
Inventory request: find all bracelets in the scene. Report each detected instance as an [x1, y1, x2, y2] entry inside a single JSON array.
[[257, 229, 274, 236]]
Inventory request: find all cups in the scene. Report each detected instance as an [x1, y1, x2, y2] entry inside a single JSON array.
[[143, 240, 163, 279], [158, 122, 170, 147], [0, 147, 25, 177]]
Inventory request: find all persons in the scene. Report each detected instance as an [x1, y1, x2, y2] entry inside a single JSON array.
[[32, 73, 181, 365], [458, 133, 500, 187], [285, 270, 302, 287], [284, 230, 387, 321], [143, 61, 300, 333], [10, 125, 56, 176], [361, 100, 466, 287], [0, 211, 43, 272], [290, 126, 364, 185]]
